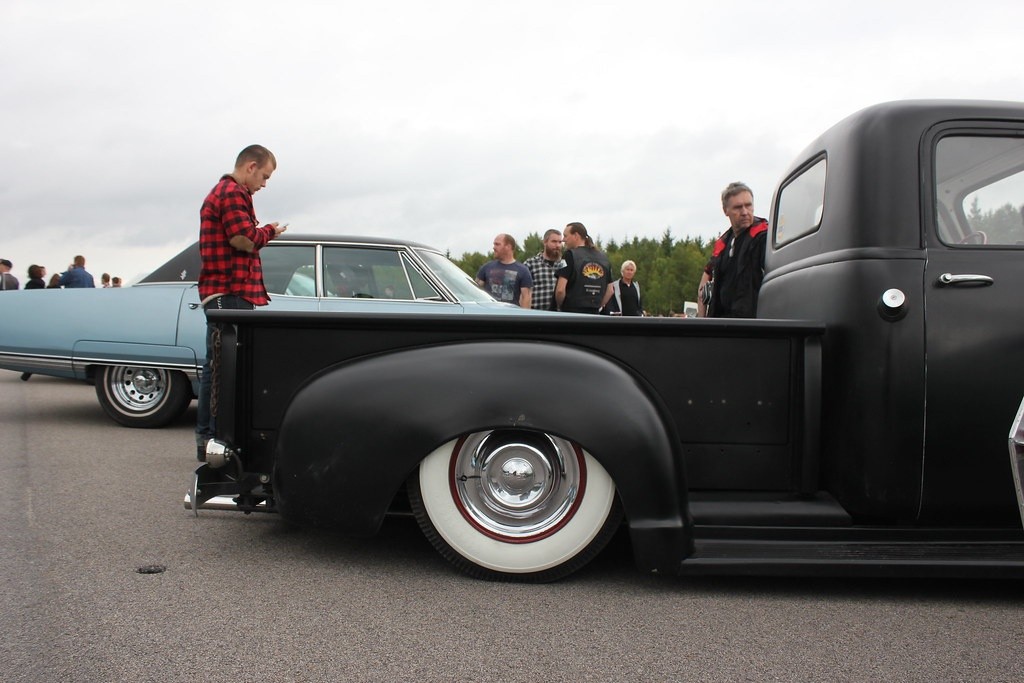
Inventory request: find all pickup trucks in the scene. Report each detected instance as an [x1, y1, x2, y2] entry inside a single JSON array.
[[189, 100, 1024, 591]]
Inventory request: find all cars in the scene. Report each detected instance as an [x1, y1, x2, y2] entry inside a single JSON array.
[[0, 231, 609, 431]]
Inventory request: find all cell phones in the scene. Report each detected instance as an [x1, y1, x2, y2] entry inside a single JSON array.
[[282, 222, 290, 228]]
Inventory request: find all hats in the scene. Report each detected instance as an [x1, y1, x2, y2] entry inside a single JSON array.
[[29, 265, 45, 271], [0, 260, 11, 267], [60, 272, 67, 274]]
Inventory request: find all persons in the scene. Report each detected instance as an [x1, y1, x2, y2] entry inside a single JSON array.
[[522, 229, 568, 311], [199, 144, 287, 433], [697, 181, 770, 318], [0, 258, 20, 290], [601, 259, 645, 316], [24, 264, 120, 289], [46, 256, 96, 288], [475, 233, 533, 308], [553, 222, 615, 314]]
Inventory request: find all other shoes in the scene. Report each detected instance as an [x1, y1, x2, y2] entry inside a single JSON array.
[[198, 446, 206, 461]]
